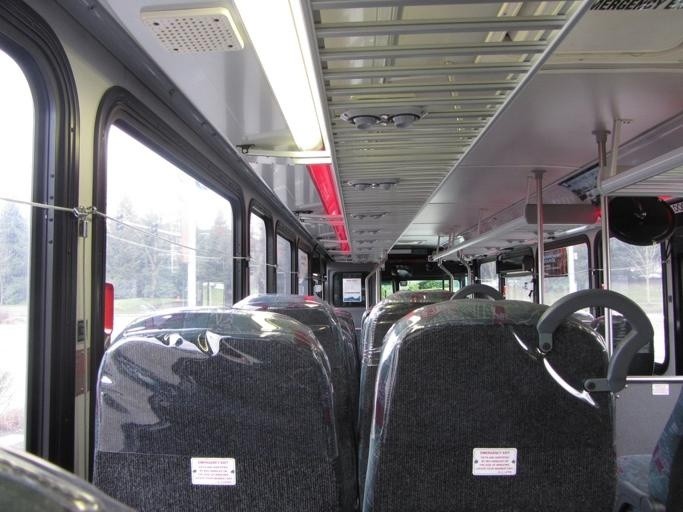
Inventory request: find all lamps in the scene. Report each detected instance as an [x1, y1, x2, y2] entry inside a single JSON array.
[[234, 0, 327, 154]]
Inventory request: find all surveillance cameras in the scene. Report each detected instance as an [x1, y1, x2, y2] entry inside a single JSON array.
[[425, 264, 434, 272]]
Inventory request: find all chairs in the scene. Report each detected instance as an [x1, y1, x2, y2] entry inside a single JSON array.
[[1, 284, 683, 512]]
